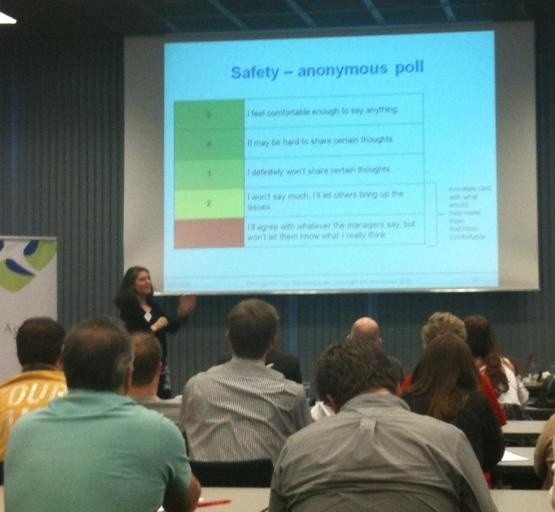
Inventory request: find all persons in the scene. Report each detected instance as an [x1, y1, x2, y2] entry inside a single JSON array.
[[533, 366, 555, 512], [1, 317, 201, 511], [113, 266, 197, 399], [180, 298, 314, 487], [268, 311, 530, 512]]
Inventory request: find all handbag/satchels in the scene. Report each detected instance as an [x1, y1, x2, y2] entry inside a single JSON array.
[[530, 375, 555, 409]]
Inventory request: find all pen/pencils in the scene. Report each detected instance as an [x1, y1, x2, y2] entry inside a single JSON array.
[[198, 500, 229, 507]]
